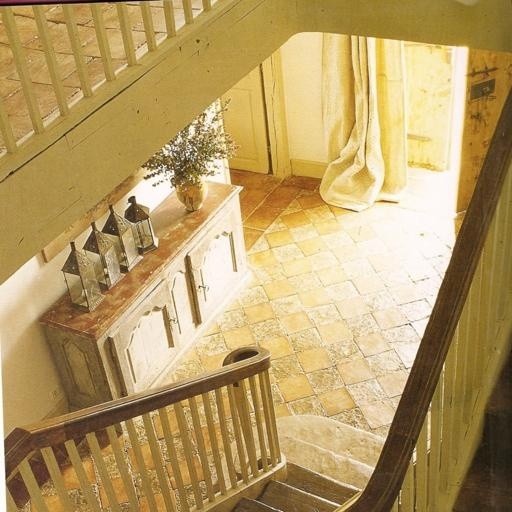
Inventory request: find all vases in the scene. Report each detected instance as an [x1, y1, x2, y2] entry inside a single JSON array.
[[176, 175, 207, 212]]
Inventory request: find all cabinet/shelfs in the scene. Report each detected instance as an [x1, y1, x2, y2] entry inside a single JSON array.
[[39, 180, 253, 432]]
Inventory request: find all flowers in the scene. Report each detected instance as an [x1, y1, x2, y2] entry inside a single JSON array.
[[140, 97, 242, 193]]
